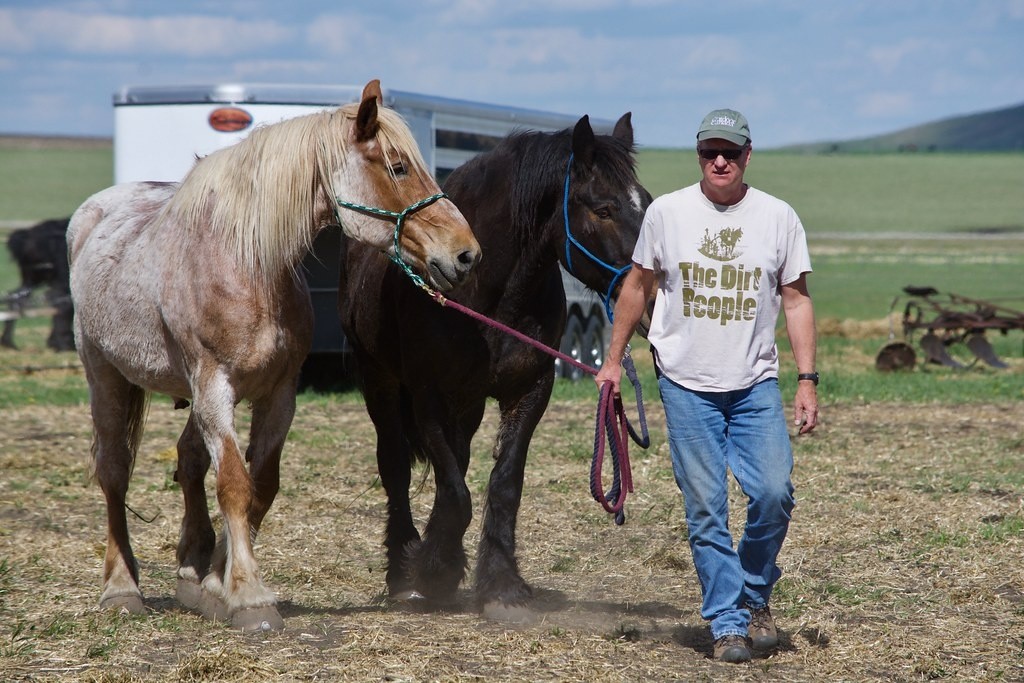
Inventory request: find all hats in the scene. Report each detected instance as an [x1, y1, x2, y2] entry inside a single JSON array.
[[696, 108, 752, 146]]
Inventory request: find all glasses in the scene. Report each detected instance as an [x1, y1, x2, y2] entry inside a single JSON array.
[[697, 146, 749, 160]]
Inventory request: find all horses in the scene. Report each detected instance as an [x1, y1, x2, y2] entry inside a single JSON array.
[[175, 112, 654, 634], [64, 77, 484, 636]]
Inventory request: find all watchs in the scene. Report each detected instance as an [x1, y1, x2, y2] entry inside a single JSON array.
[[799, 372, 819, 386]]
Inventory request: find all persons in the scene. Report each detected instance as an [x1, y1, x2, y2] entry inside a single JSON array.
[[595, 109, 820, 664]]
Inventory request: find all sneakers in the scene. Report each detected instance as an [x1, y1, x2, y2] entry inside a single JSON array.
[[746, 605, 779, 650], [712, 635, 751, 664]]
